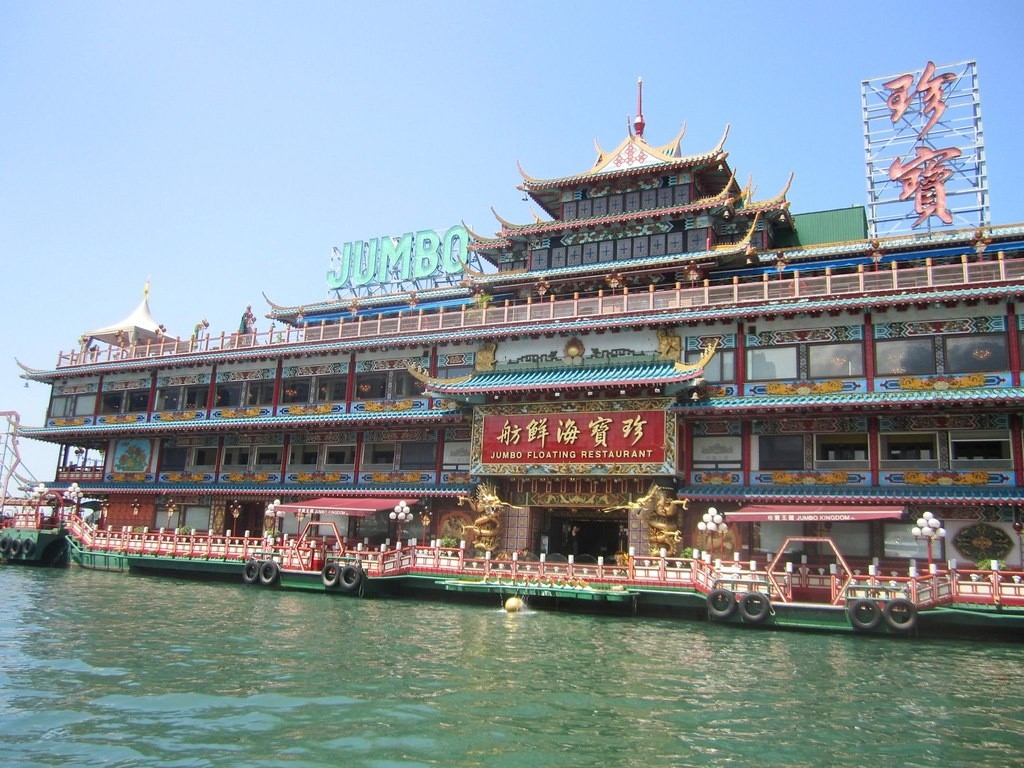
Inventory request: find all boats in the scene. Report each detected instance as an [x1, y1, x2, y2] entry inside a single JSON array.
[[2, 58, 1024, 643]]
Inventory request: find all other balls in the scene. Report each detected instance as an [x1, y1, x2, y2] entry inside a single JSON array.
[[505, 598, 523, 613]]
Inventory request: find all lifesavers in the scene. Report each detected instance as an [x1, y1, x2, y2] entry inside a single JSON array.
[[339, 566, 364, 589], [849, 597, 882, 630], [259, 561, 278, 586], [22, 537, 35, 555], [705, 588, 735, 618], [883, 598, 919, 632], [9, 538, 22, 557], [243, 560, 260, 583], [0, 536, 10, 554], [321, 562, 340, 588], [738, 590, 768, 622]]
